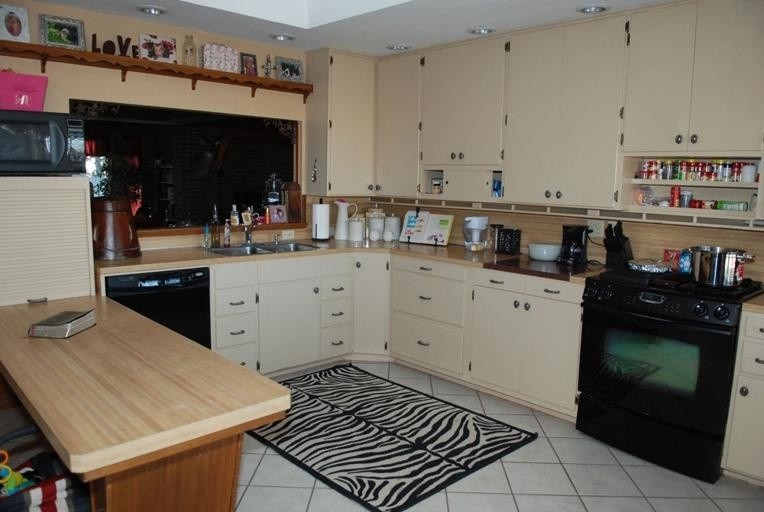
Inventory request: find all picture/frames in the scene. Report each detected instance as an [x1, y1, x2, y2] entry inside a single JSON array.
[[39, 14, 85, 50], [240, 53, 257, 76], [275, 56, 304, 82], [0, 5, 30, 43]]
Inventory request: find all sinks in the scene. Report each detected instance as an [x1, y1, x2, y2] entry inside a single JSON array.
[[210, 245, 276, 256], [261, 243, 320, 253]]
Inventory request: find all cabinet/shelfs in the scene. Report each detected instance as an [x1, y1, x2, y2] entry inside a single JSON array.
[[720, 311, 764, 488]]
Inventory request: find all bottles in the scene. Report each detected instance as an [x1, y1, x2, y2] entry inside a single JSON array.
[[203, 203, 239, 249], [670, 185, 747, 212], [489, 223, 504, 253], [265, 208, 270, 224], [182, 34, 197, 67], [639, 160, 757, 182], [431, 177, 443, 194], [492, 173, 501, 197], [332, 201, 358, 240]]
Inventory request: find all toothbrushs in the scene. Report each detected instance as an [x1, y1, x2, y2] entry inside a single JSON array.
[[247, 206, 253, 213]]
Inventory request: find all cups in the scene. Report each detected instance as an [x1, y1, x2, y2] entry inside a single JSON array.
[[349, 221, 363, 242], [369, 231, 380, 242], [383, 230, 393, 242]]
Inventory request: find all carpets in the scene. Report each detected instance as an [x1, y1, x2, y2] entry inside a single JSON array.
[[245, 364, 537, 512]]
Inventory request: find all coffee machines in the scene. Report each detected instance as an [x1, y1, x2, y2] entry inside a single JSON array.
[[557, 224, 593, 265]]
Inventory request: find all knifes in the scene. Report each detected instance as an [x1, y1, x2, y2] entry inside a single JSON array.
[[604, 222, 626, 242]]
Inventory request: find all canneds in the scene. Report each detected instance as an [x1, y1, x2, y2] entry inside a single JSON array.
[[640, 158, 756, 182], [671, 186, 680, 207]]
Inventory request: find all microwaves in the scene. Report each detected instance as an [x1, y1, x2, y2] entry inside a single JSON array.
[[0, 109, 86, 177]]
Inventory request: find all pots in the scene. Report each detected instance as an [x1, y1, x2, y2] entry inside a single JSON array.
[[681, 244, 756, 289]]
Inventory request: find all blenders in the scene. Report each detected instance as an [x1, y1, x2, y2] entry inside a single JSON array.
[[462, 216, 489, 252]]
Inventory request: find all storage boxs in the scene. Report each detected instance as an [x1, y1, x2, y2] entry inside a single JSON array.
[[0, 72, 48, 112]]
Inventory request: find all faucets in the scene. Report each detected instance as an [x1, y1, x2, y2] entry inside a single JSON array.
[[243, 223, 256, 245], [274, 232, 280, 244]]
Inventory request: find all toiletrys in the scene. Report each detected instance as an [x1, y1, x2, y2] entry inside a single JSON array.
[[224, 219, 231, 248], [231, 205, 239, 226], [201, 223, 211, 247]]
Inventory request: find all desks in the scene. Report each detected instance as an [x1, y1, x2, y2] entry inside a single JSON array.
[[1, 295, 291, 512]]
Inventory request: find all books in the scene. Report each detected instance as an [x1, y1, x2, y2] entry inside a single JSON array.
[[398, 210, 455, 246], [28, 308, 98, 340]]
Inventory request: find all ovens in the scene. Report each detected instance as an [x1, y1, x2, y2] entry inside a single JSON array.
[[105, 267, 211, 352], [576, 277, 740, 484]]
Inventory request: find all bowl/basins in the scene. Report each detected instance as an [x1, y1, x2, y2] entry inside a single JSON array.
[[527, 243, 561, 262], [528, 261, 559, 274]]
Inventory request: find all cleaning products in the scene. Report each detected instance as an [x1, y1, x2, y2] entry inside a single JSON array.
[[211, 215, 220, 248]]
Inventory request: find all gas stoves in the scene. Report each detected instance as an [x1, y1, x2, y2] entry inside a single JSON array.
[[585, 261, 763, 304]]
